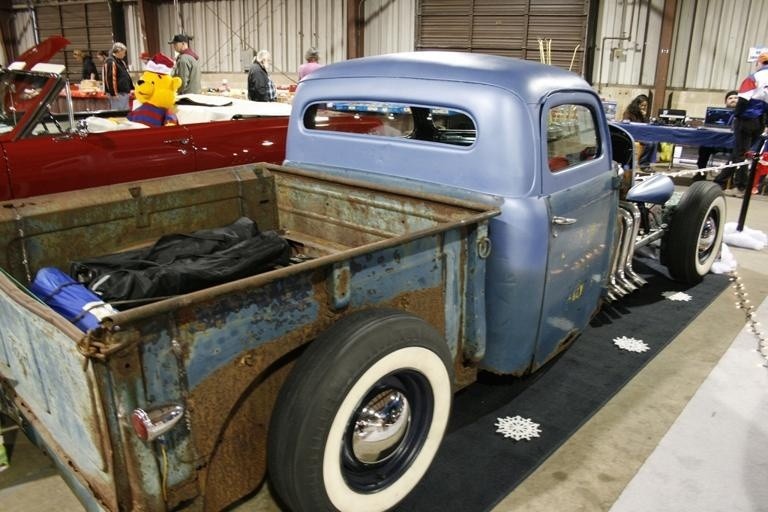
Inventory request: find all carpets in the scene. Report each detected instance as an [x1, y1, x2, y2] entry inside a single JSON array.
[[379, 268, 742, 512]]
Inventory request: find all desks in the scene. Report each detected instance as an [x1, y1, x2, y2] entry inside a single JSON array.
[[605, 120, 767, 193]]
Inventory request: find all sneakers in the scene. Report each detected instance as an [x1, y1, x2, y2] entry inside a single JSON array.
[[724, 187, 746, 197], [693, 172, 707, 180], [642, 167, 655, 173]]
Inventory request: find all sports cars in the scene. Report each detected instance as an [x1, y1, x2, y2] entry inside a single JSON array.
[[0, 32, 389, 202]]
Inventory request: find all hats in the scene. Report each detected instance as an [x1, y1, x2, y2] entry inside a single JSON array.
[[167, 34, 187, 44], [758, 52, 768, 63]]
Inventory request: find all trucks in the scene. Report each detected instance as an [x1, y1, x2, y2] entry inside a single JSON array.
[[0, 49, 725, 512]]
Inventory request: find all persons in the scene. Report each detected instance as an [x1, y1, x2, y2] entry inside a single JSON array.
[[72, 48, 99, 80], [102, 41, 134, 111], [167, 34, 203, 95], [621, 95, 656, 175], [96, 51, 107, 79], [297, 46, 326, 82], [688, 92, 739, 184], [247, 50, 276, 103], [712, 51, 768, 198]]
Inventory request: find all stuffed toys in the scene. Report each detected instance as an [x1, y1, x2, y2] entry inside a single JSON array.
[[107, 54, 183, 129]]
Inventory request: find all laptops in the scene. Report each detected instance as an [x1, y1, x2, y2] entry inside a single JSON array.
[[658, 110, 685, 125], [704, 107, 736, 128]]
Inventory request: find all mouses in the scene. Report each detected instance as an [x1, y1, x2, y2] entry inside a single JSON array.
[[698, 126, 707, 130]]
